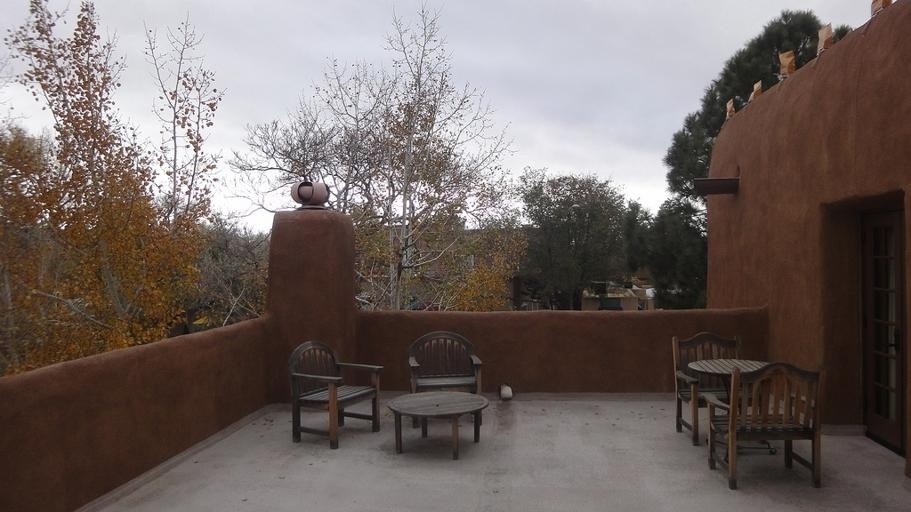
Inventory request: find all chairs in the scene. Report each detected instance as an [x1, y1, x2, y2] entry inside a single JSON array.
[[408, 330, 484, 429], [287, 340, 385, 449], [673, 331, 827, 490]]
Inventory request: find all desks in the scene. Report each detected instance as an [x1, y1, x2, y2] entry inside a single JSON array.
[[386, 391, 490, 460]]
